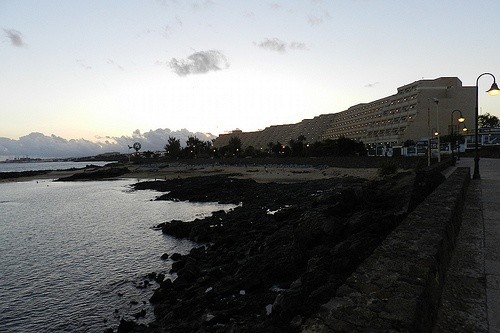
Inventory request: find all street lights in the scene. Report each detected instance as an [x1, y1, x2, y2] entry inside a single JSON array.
[[457, 122, 467, 161], [451, 110, 465, 166], [472, 73, 500, 179], [430, 127, 438, 167]]
